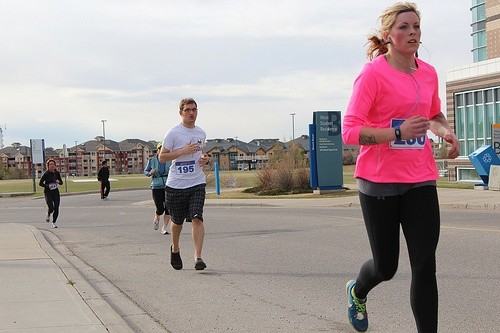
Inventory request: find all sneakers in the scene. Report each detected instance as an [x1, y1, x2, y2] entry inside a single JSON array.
[[346, 280, 368, 333], [171, 244, 183, 270], [194, 257, 206, 270]]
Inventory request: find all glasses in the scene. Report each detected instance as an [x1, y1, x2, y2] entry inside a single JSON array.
[[181, 108, 198, 112]]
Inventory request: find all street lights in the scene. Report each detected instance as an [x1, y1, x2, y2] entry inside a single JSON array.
[[101, 119, 108, 161], [290, 113, 296, 141]]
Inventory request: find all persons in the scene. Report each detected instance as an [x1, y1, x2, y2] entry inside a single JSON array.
[[97, 161, 110, 200], [342, 0, 460, 333], [144, 143, 172, 234], [160, 98, 209, 270], [40, 159, 63, 228]]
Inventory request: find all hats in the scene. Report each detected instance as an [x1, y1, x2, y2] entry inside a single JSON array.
[[157, 142, 162, 149]]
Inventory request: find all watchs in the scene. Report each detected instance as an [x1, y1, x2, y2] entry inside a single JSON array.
[[394, 126, 402, 141]]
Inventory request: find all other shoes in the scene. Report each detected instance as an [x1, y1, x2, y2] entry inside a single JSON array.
[[52, 223, 57, 228], [153, 217, 159, 230], [162, 228, 169, 234], [46, 214, 50, 222]]
